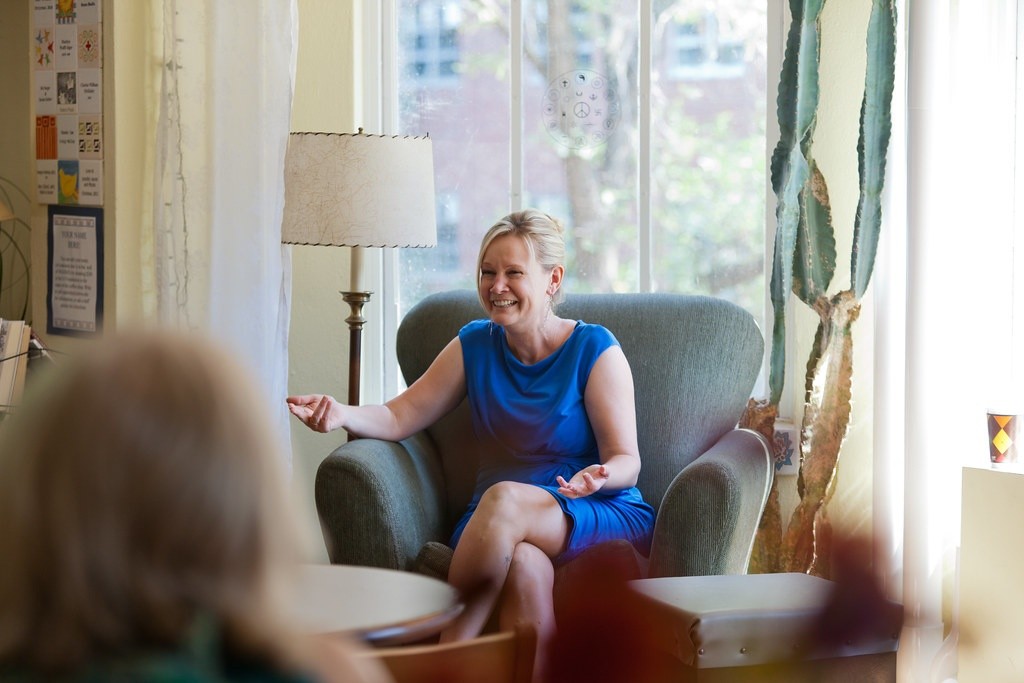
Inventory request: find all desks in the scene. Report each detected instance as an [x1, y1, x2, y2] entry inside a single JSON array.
[[264, 563, 466, 646]]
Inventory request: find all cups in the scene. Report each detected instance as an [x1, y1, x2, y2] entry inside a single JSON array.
[[988, 412, 1017, 465]]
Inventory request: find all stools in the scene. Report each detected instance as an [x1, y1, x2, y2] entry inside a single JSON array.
[[619, 572, 904, 683]]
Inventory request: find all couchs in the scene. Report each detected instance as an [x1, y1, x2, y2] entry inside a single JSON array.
[[315, 287, 786, 580]]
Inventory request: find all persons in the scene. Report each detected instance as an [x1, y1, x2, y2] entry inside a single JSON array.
[[287, 208, 656, 683], [0, 323, 392, 683]]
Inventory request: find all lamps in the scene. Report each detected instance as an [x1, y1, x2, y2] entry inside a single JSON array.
[[282, 126, 438, 444]]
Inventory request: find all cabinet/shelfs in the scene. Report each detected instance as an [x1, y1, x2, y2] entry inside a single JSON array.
[[960, 462, 1024, 683]]
